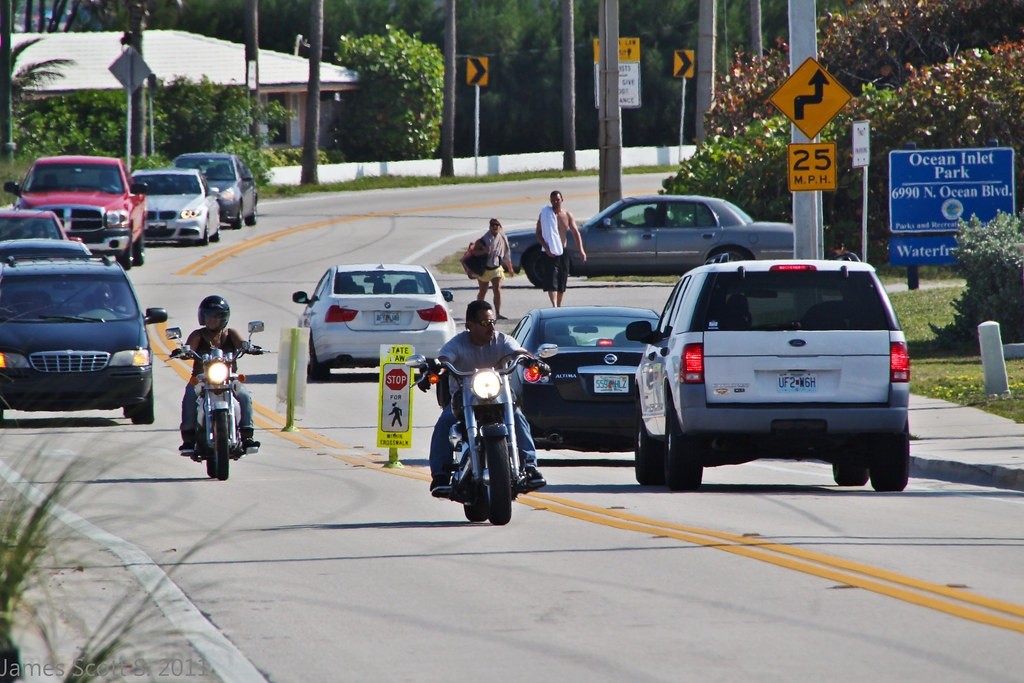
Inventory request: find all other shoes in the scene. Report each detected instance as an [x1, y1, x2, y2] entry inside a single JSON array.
[[429, 477, 448, 490], [526, 468, 545, 484], [496, 315, 508, 319]]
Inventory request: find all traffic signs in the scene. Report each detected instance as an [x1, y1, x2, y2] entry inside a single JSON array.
[[595, 62, 642, 109]]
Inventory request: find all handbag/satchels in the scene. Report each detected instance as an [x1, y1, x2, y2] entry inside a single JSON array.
[[461, 240, 488, 279]]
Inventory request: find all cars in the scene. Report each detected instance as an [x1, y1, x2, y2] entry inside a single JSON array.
[[499, 195, 794, 289], [124, 152, 259, 247], [0, 210, 83, 242], [293, 263, 458, 382], [506, 304, 661, 453]]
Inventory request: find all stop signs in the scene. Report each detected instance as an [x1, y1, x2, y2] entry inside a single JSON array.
[[386, 368, 408, 390]]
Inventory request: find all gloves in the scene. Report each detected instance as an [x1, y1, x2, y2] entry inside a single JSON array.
[[418, 375, 431, 393], [170, 348, 182, 358], [533, 359, 551, 377], [251, 346, 262, 355]]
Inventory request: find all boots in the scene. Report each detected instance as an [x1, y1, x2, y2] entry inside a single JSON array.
[[179, 430, 196, 450], [240, 427, 260, 450]]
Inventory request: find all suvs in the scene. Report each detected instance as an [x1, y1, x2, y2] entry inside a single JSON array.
[[627, 257, 913, 492], [0, 253, 169, 425], [3, 156, 149, 270]]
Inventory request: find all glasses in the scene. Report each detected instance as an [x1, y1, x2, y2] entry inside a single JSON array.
[[492, 224, 501, 227], [470, 318, 497, 326]]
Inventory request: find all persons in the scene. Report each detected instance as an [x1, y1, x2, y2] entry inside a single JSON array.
[[616, 206, 656, 227], [536, 191, 587, 307], [171, 295, 263, 453], [89, 285, 127, 314], [478, 218, 514, 319], [429, 300, 545, 498]]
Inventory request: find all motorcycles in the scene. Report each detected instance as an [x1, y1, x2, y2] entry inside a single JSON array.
[[402, 343, 562, 526], [164, 321, 272, 482]]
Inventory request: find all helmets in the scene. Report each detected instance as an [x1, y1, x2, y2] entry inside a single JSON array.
[[198, 295, 230, 329]]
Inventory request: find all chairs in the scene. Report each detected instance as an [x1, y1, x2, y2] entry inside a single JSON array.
[[550, 334, 577, 347], [43, 174, 56, 189], [342, 279, 417, 294]]
